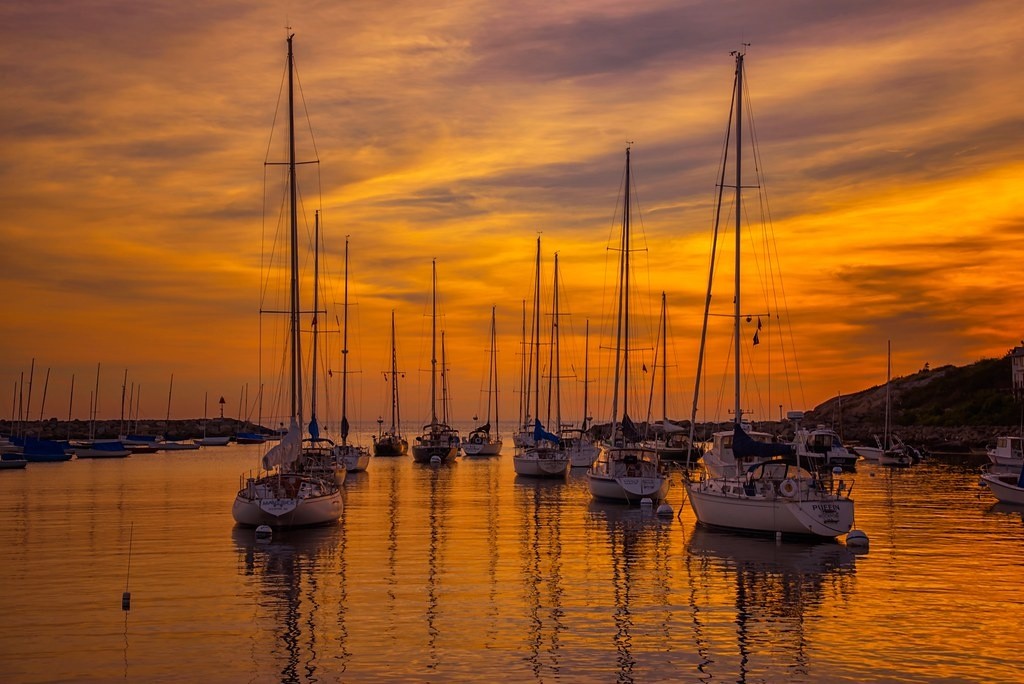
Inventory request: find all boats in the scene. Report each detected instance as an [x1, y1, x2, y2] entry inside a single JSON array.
[[853, 443, 923, 460], [986, 436, 1024, 464], [980, 463, 1024, 505], [777, 425, 858, 470]]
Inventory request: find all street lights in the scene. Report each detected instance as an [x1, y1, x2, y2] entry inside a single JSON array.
[[779, 405, 782, 420]]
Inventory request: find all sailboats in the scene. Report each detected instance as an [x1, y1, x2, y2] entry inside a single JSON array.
[[553, 253, 602, 466], [229, 382, 280, 444], [512, 299, 545, 448], [879, 340, 912, 468], [461, 306, 503, 455], [673, 49, 856, 537], [512, 237, 572, 478], [193, 392, 230, 446], [0, 358, 200, 468], [639, 291, 706, 465], [586, 148, 673, 505], [233, 40, 343, 531], [412, 261, 461, 464], [373, 309, 408, 457], [280, 210, 371, 484]]
